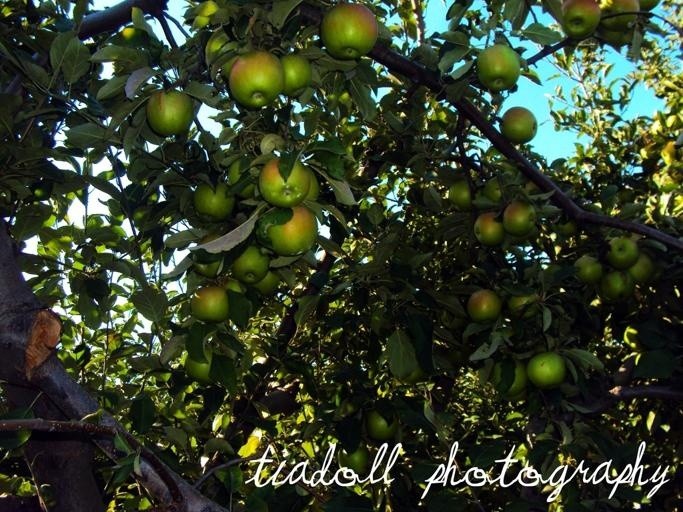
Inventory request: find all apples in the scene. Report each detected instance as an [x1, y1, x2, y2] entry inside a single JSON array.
[[99, 0, 657, 479]]
